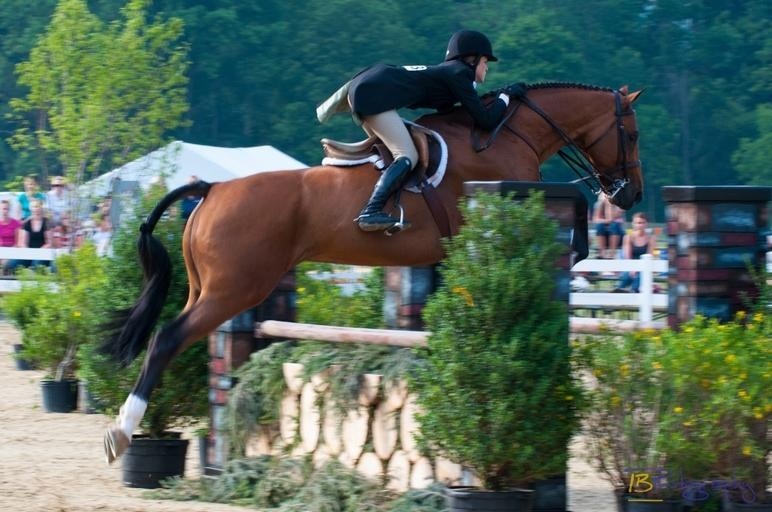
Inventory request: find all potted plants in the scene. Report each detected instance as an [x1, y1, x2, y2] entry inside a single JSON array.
[[423, 184, 593, 512]]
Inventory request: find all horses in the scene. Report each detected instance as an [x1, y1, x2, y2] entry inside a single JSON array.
[[87, 81, 647, 464]]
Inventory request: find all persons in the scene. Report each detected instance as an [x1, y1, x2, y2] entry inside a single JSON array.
[[346, 29, 511, 232], [592, 190, 625, 259], [0, 172, 203, 278], [616, 212, 656, 293]]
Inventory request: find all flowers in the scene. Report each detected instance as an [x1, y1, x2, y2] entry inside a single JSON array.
[[564, 309, 772, 496]]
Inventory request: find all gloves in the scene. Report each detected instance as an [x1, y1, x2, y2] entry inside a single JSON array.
[[503, 82, 528, 100]]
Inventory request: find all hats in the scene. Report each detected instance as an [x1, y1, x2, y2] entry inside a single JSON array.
[[445, 29, 498, 62]]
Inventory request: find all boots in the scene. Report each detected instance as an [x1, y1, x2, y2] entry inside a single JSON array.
[[357, 156, 412, 233]]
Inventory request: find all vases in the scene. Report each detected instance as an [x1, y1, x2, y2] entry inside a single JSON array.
[[611, 486, 713, 512]]
